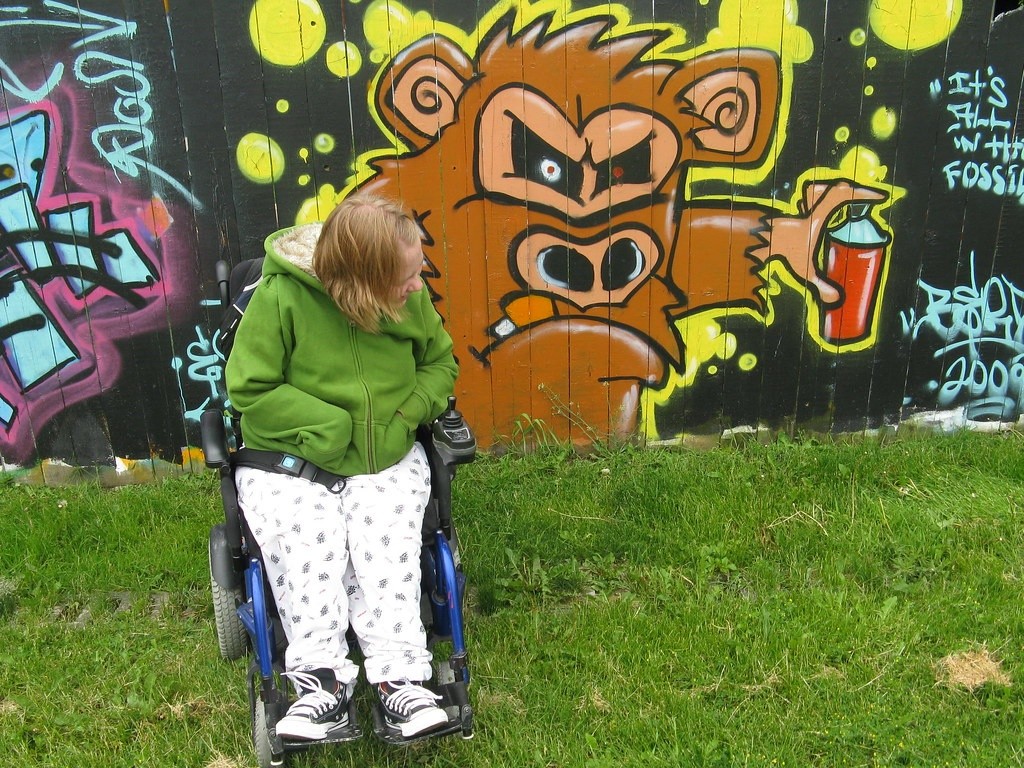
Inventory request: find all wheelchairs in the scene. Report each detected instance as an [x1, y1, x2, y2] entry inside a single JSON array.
[[196, 256, 480, 768]]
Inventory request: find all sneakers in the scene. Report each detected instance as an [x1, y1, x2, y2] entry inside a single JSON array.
[[376, 678, 448, 737], [276, 667, 349, 740]]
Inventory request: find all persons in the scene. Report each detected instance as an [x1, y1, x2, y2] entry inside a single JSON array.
[[224, 196, 457, 739]]
